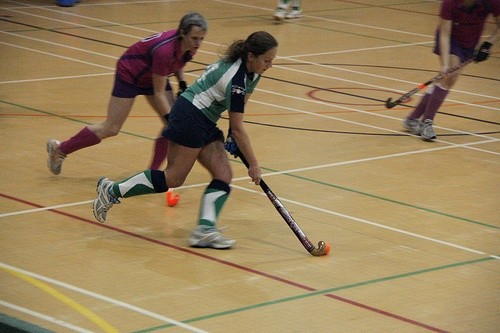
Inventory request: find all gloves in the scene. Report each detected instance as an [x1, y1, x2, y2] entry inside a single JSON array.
[[473, 41, 493, 63], [224, 135, 239, 159], [176, 81, 188, 98]]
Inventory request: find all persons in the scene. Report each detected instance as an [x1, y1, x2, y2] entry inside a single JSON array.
[[403, 0, 500, 142], [47, 12, 208, 176], [273, 0, 303, 19], [93, 31, 278, 249]]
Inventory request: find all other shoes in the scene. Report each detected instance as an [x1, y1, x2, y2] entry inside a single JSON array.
[[286, 6, 302, 18], [273, 4, 288, 20]]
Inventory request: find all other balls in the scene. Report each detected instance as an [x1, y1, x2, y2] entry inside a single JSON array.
[[323, 242, 331, 255]]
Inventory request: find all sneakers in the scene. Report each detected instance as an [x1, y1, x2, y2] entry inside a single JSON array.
[[46, 139, 67, 176], [188, 227, 236, 249], [403, 118, 422, 135], [414, 118, 438, 140], [92, 177, 121, 225]]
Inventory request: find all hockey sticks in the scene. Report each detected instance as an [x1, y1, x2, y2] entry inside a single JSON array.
[[385, 56, 477, 109], [166, 187, 180, 207], [226, 133, 326, 256]]
